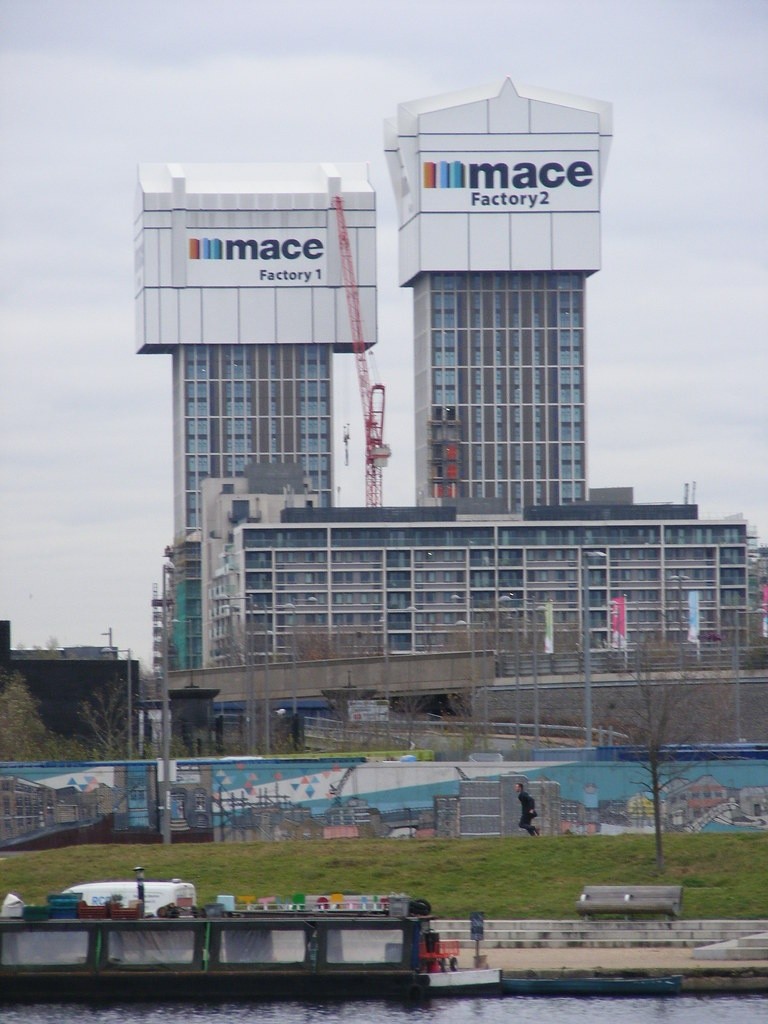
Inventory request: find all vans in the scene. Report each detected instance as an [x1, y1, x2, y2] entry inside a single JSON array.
[[59, 875, 198, 918]]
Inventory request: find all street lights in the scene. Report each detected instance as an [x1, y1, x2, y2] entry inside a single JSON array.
[[498, 595, 538, 749], [101, 646, 133, 760], [512, 606, 550, 748], [263, 603, 297, 754], [608, 600, 622, 652], [668, 573, 691, 671], [291, 596, 319, 750], [732, 608, 767, 742], [580, 549, 607, 748], [407, 607, 429, 652], [170, 617, 193, 686], [213, 593, 258, 754], [159, 561, 176, 845], [449, 593, 478, 722], [452, 618, 489, 753]]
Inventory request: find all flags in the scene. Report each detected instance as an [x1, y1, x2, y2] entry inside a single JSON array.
[[544, 604, 554, 654], [611, 595, 626, 640], [687, 591, 700, 644], [763, 583, 768, 636]]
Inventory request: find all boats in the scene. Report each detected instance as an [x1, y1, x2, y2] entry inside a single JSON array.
[[498, 971, 687, 1000], [0, 866, 506, 1002]]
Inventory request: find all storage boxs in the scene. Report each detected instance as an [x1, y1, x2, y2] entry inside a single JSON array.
[[21, 905, 50, 921], [47, 894, 80, 919], [204, 902, 224, 917]]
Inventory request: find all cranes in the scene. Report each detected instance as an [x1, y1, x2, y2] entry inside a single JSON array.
[[327, 193, 394, 507]]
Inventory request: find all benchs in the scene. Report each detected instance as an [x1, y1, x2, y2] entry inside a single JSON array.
[[574, 885, 684, 920]]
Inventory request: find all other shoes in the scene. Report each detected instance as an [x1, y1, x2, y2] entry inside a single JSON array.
[[535, 828, 540, 835]]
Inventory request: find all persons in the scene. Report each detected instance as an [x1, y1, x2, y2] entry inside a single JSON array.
[[515, 783, 541, 837]]
